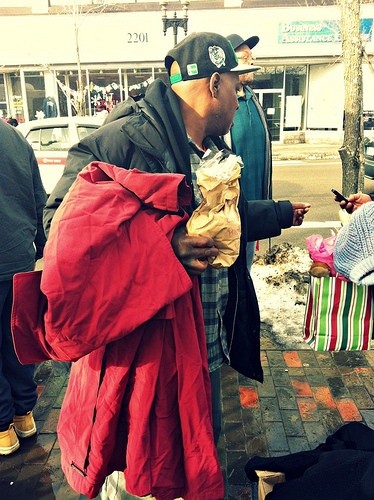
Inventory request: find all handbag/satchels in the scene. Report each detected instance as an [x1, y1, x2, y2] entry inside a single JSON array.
[[304, 264, 374, 353]]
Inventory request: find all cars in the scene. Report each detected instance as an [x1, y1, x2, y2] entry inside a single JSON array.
[[15, 117, 104, 196]]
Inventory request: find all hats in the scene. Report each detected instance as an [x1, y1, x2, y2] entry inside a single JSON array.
[[165, 32, 261, 84], [333, 201, 374, 285], [226, 34, 259, 52]]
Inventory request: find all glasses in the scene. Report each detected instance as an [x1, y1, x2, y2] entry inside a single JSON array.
[[237, 55, 257, 63]]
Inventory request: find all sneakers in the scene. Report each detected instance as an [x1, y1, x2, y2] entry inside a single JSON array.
[[1, 423, 20, 455], [13, 411, 36, 438]]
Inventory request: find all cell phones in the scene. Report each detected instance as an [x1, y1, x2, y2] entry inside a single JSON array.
[[331, 189, 354, 207]]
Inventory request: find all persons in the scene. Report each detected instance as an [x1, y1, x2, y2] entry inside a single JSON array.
[[43, 33, 311, 500], [334, 192, 374, 213], [7, 115, 18, 127], [0, 120, 47, 455], [218, 34, 271, 274]]
